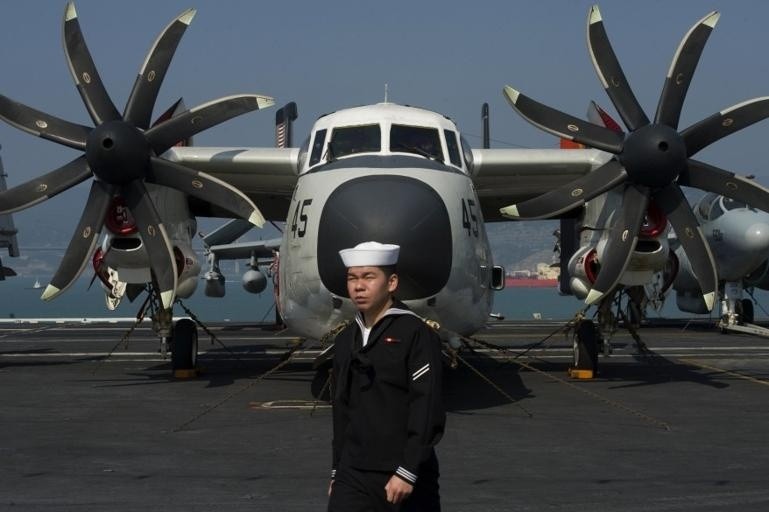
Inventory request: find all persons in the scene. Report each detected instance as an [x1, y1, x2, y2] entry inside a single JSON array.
[[327, 240, 446, 512]]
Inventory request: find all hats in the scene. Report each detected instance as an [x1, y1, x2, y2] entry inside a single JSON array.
[[338, 241, 400, 268]]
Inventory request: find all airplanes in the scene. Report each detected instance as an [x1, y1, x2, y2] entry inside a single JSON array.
[[0, 0, 768, 380]]
